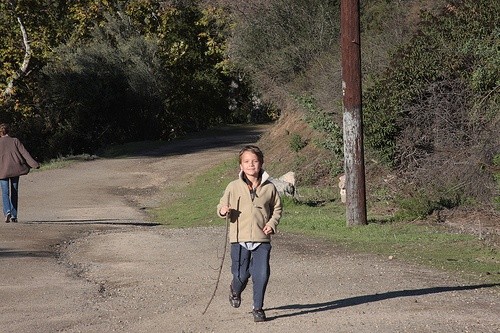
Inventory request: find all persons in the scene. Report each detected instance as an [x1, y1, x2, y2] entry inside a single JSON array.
[[216, 146, 283, 323], [0, 122, 41, 222]]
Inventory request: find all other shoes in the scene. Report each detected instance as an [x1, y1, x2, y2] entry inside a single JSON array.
[[10, 215, 17, 222], [4, 212, 11, 223]]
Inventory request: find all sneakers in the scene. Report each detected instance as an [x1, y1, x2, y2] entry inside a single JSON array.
[[229, 285, 242, 308], [252, 306, 266, 322]]
